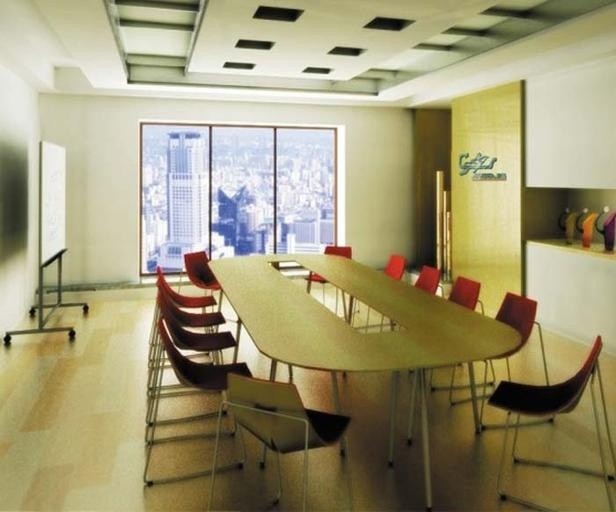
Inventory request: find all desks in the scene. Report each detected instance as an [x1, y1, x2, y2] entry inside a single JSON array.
[[205, 254, 521, 505]]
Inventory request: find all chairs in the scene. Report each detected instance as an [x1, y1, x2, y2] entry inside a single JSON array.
[[207, 372, 358, 510], [386, 265, 442, 335], [406, 278, 497, 392], [177, 251, 238, 324], [349, 255, 407, 333], [485, 336, 616, 512], [448, 292, 557, 438], [306, 246, 359, 319], [153, 266, 228, 388], [145, 318, 255, 485], [145, 301, 237, 439]]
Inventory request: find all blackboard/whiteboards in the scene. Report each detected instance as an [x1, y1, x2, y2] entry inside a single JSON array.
[[39, 141, 67, 268]]
